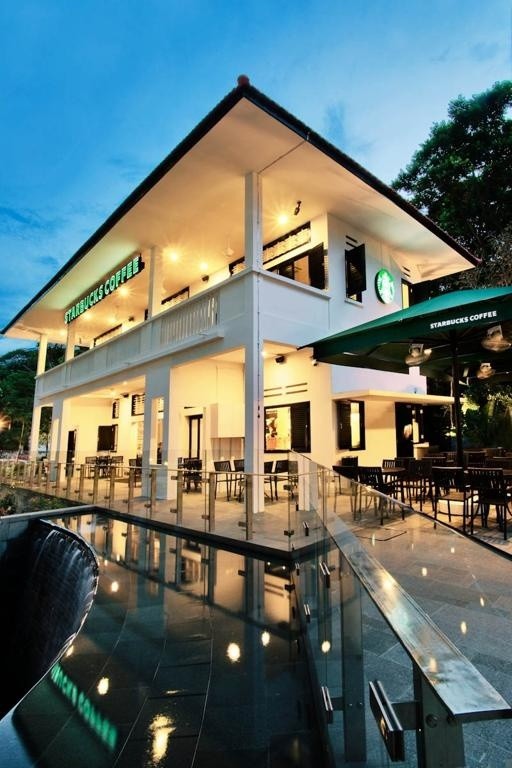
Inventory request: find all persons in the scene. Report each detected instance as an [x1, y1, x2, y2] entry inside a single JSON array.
[[158, 441, 162, 464]]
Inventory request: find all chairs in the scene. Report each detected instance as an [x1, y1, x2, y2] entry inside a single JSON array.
[[177, 457, 203, 494], [213, 459, 298, 502], [332, 446, 512, 540], [136, 456, 142, 471], [85, 455, 124, 478], [128, 459, 142, 489]]
